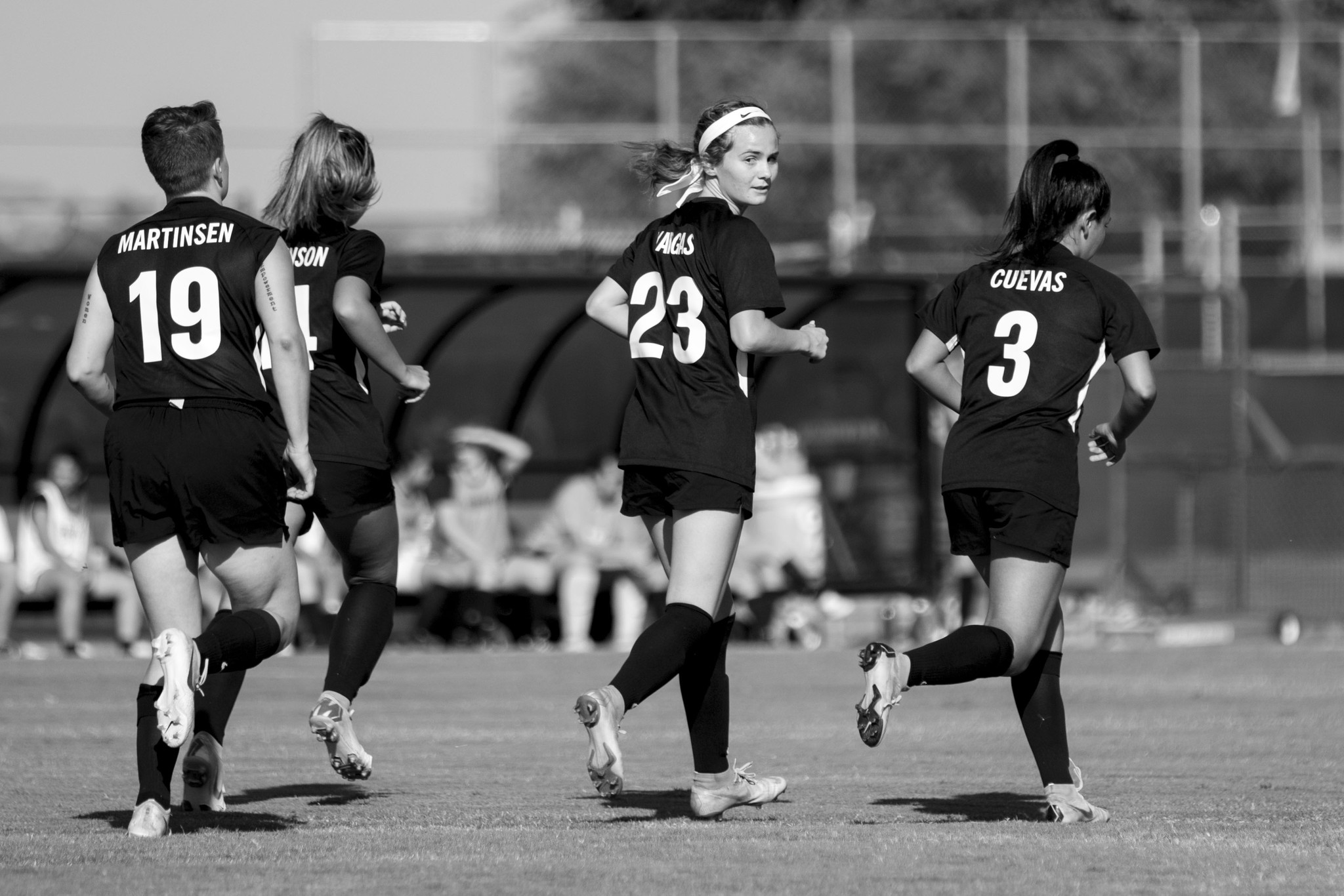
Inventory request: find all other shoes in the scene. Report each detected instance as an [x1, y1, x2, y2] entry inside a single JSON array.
[[817, 587, 858, 623], [787, 614, 823, 653]]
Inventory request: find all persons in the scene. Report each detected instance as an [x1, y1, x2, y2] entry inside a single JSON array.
[[63, 100, 319, 841], [0, 423, 832, 658], [177, 111, 433, 817], [853, 139, 1160, 827], [574, 101, 830, 820]]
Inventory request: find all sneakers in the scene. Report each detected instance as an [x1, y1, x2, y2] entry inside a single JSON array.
[[689, 757, 788, 820], [151, 627, 210, 748], [308, 695, 375, 782], [854, 641, 911, 749], [126, 798, 173, 838], [573, 684, 625, 800], [1044, 756, 1111, 824], [178, 730, 227, 814]]
